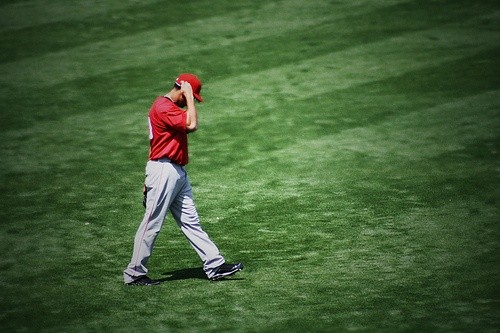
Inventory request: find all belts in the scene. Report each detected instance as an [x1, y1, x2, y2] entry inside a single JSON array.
[[156, 159, 177, 163]]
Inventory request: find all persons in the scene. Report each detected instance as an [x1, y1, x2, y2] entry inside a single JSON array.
[[123, 74, 244, 286]]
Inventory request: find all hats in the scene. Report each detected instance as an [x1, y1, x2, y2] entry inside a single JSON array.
[[175, 73, 203, 103]]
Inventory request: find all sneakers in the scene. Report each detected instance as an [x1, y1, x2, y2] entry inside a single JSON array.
[[211, 263, 241, 281], [129, 275, 160, 287]]
